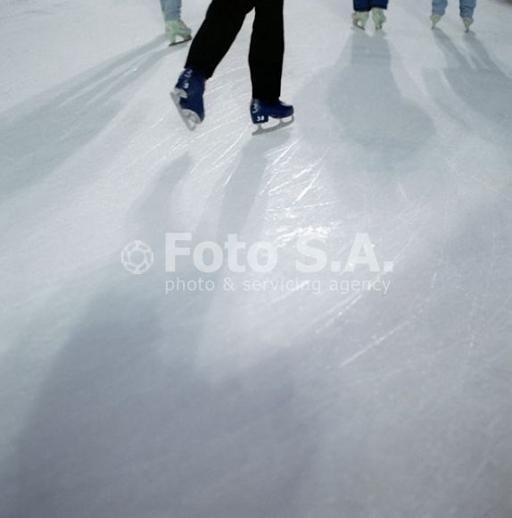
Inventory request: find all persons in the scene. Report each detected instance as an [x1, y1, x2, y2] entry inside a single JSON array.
[[430, 0, 477, 27], [351, 0, 389, 25], [175, 0, 294, 124], [158, 0, 192, 43]]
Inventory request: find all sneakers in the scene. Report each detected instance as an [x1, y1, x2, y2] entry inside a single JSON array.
[[464, 17, 473, 27], [250, 98, 294, 125], [164, 20, 191, 36], [175, 69, 204, 124], [352, 8, 386, 24], [430, 14, 441, 22]]
